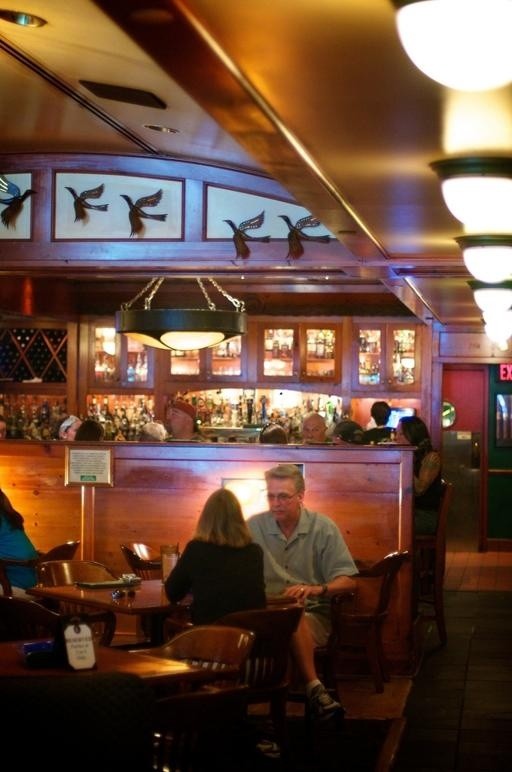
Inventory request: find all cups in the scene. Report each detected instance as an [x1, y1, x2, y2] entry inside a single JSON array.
[[161, 545, 182, 584]]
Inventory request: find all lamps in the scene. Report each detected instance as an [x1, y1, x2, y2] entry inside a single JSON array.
[[116, 277, 245, 351], [432, 158, 512, 226], [467, 280, 512, 311], [451, 233, 512, 282]]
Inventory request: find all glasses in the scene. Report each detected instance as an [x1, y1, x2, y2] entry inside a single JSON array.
[[266, 489, 300, 500]]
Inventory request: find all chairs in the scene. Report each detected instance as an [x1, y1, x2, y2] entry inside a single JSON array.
[[207, 605, 305, 724], [129, 626, 256, 772], [325, 551, 408, 694], [412, 479, 456, 645], [120, 542, 163, 580], [36, 560, 151, 651], [0, 538, 80, 604]]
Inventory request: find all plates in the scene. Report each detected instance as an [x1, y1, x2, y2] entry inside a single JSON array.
[[163, 437, 201, 443], [74, 574, 144, 590], [377, 441, 411, 448], [309, 441, 339, 447]]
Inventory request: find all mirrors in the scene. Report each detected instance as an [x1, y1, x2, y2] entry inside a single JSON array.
[[441, 400, 457, 429], [494, 391, 512, 450]]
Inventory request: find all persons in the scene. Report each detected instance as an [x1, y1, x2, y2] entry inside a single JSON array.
[[140, 423, 166, 441], [1, 491, 48, 601], [166, 488, 264, 628], [393, 417, 444, 534], [366, 403, 397, 444], [261, 424, 290, 444], [55, 415, 81, 440], [301, 413, 328, 443], [75, 420, 106, 440], [165, 402, 206, 443], [333, 421, 365, 444], [242, 463, 362, 759]]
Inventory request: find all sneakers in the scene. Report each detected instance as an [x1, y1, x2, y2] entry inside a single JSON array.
[[256, 717, 283, 759], [305, 679, 344, 723]]
